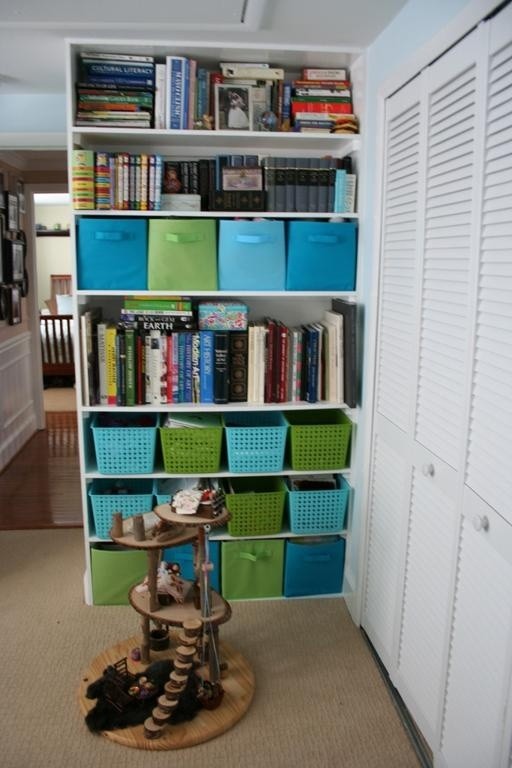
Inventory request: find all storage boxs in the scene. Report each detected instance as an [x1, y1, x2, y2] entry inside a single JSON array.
[[78, 217, 357, 291], [92, 536, 345, 605]]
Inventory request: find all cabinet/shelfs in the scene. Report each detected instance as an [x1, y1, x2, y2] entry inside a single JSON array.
[[64, 39, 367, 606]]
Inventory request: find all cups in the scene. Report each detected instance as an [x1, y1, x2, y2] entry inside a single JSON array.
[[53, 223, 60, 229]]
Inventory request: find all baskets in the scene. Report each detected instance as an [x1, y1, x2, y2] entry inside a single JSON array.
[[158, 412, 226, 474], [282, 409, 352, 470], [285, 474, 352, 534], [89, 479, 155, 539], [219, 476, 286, 537], [224, 411, 290, 473], [155, 478, 219, 506], [90, 413, 160, 474]]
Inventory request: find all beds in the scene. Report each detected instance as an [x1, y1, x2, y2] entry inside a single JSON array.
[[40, 274, 73, 375]]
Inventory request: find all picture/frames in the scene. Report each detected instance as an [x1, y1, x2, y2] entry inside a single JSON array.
[[214, 84, 253, 131], [0, 190, 25, 326]]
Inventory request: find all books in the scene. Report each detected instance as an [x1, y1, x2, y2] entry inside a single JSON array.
[[74, 48, 358, 214], [81, 294, 360, 409], [163, 411, 222, 460]]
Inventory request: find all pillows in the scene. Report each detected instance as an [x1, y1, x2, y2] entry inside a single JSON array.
[[56, 294, 73, 315]]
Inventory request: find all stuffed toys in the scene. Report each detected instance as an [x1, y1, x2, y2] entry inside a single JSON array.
[[170, 487, 224, 519], [136, 560, 191, 604], [82, 655, 224, 731]]
